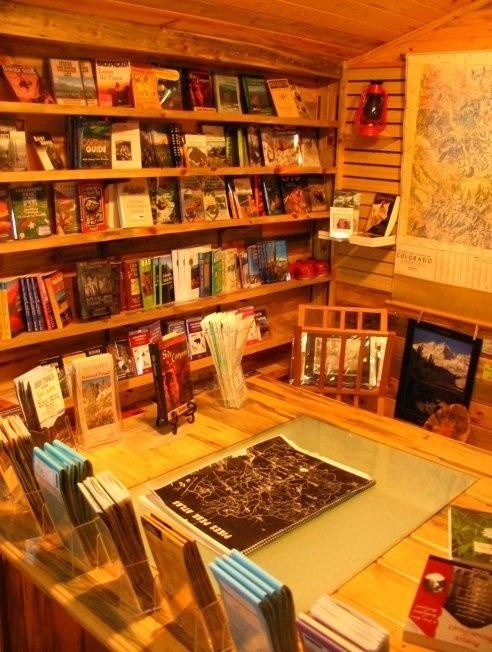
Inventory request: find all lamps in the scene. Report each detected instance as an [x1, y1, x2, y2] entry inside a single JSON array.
[[354, 79, 387, 139]]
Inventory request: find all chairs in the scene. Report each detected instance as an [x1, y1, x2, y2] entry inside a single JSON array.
[[295, 303, 396, 416]]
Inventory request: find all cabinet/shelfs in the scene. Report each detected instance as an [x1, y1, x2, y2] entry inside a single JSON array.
[[1, 28, 343, 436]]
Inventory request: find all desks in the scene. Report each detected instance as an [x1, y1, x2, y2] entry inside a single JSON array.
[[0, 370, 492, 652]]
